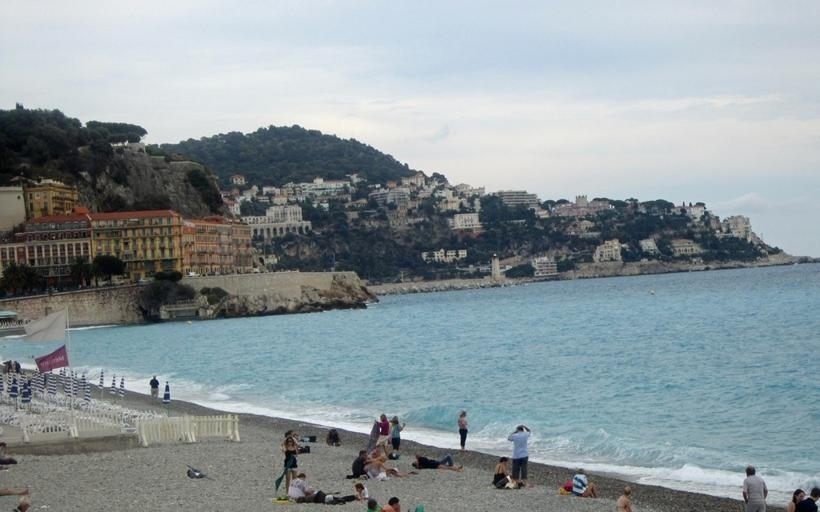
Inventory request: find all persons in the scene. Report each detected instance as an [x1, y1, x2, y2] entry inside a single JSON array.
[[10, 497, 31, 512], [794, 486, 820, 511], [3, 360, 20, 374], [508, 425, 531, 481], [493, 457, 511, 489], [616, 486, 632, 512], [411, 453, 464, 472], [457, 409, 468, 450], [789, 489, 806, 512], [281, 414, 426, 512], [743, 465, 768, 512], [572, 468, 596, 499], [150, 375, 160, 401]]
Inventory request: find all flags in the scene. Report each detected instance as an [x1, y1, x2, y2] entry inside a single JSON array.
[[34, 344, 69, 373], [23, 309, 67, 341]]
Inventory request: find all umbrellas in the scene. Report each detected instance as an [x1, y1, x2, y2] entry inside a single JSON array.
[[0, 367, 91, 412], [162, 380, 173, 407], [109, 374, 115, 395], [99, 369, 104, 402], [118, 375, 124, 398]]
